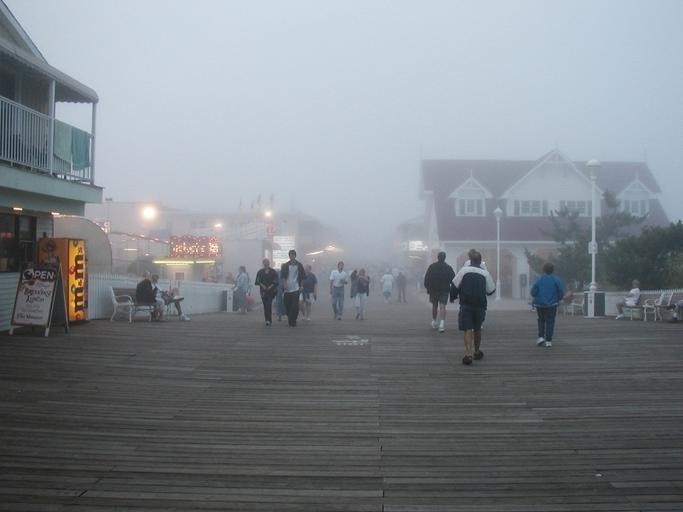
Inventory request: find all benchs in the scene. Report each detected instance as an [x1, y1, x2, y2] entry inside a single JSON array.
[[106, 285, 175, 322], [529, 291, 683, 321]]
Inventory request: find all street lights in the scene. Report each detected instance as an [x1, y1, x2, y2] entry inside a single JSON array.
[[493, 204, 505, 299], [586, 156, 599, 292]]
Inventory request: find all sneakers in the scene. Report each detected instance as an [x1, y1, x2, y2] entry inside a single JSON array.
[[546, 342, 552, 348], [474, 351, 483, 360], [431, 319, 439, 329], [536, 337, 545, 345], [439, 325, 446, 332], [172, 296, 184, 302], [462, 355, 472, 364], [179, 313, 189, 322]]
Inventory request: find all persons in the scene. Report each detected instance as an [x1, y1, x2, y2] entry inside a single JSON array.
[[424, 252, 455, 332], [616, 280, 640, 319], [450, 252, 496, 364], [463, 249, 488, 331], [531, 263, 563, 346], [560, 284, 573, 304], [136, 250, 407, 326]]
